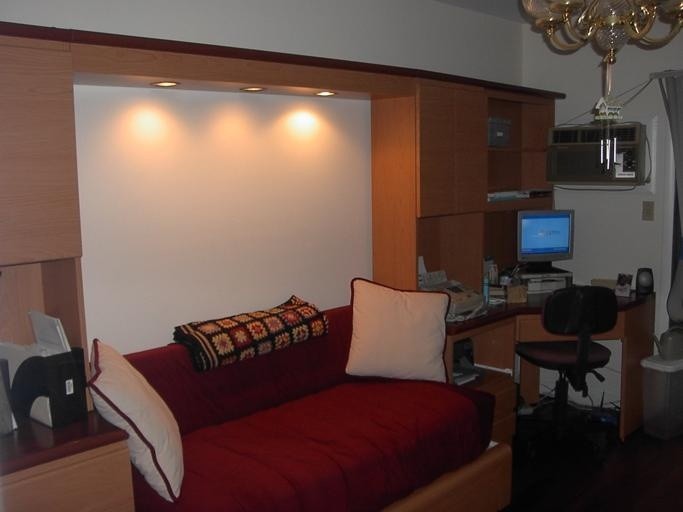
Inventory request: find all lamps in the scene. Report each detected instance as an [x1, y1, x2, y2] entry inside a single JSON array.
[[520, 0, 683, 96]]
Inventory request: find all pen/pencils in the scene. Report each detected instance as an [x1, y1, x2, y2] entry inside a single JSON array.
[[509, 264, 521, 276]]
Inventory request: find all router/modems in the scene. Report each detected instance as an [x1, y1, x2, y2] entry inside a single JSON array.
[[591, 392, 617, 423]]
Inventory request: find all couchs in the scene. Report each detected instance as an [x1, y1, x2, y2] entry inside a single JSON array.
[[89, 304, 512, 511]]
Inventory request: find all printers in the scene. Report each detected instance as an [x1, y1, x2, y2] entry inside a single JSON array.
[[419, 269, 481, 316]]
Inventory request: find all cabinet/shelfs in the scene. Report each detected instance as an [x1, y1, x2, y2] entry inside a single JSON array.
[[0, 410, 136, 512], [371, 84, 655, 444]]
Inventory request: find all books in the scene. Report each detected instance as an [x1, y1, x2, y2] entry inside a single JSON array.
[[482, 256, 528, 306]]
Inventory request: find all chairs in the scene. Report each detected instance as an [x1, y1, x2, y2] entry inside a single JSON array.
[[516, 286, 618, 465]]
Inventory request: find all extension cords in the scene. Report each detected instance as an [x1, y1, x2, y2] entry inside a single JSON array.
[[517, 404, 533, 416]]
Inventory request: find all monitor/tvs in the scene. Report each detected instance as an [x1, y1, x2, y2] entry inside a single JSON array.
[[517, 209, 574, 273]]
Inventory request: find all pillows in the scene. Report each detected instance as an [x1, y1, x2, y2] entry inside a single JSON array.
[[342, 277, 452, 385], [88, 338, 185, 504]]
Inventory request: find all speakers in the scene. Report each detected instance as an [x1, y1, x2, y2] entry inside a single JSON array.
[[636, 268, 654, 295]]
